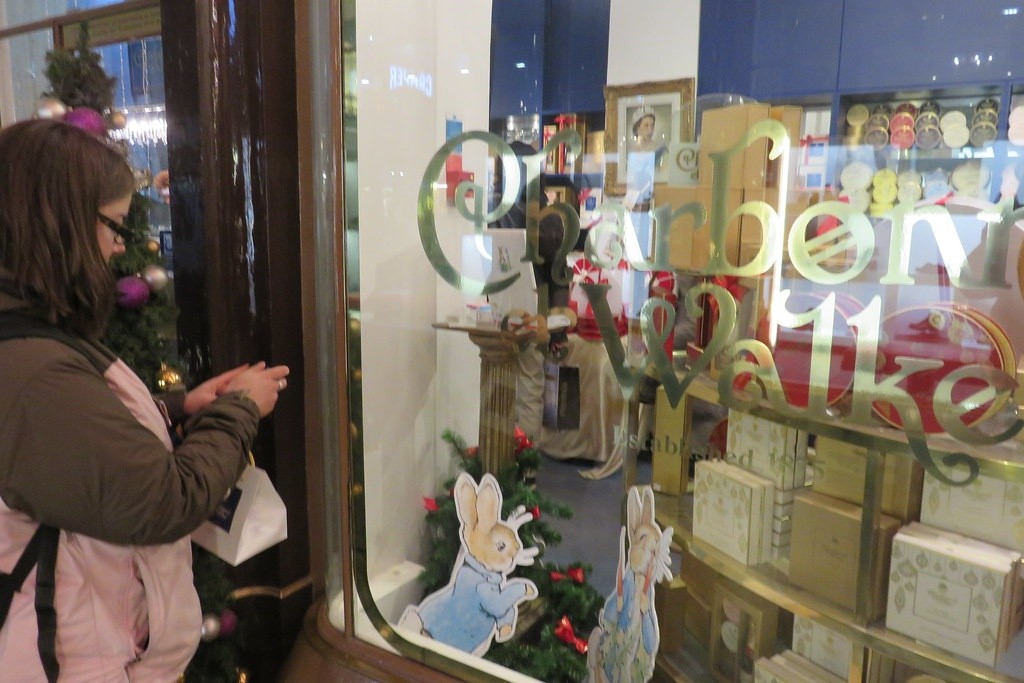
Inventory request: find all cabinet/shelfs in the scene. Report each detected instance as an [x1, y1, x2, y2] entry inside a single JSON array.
[[628, 366, 1024, 683], [819, 76, 1024, 212]]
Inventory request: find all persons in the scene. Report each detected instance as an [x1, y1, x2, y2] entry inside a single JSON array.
[[1, 119, 287, 683], [623, 106, 669, 182], [488, 144, 574, 482]]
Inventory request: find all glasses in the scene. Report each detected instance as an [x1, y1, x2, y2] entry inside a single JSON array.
[[98, 212, 136, 248]]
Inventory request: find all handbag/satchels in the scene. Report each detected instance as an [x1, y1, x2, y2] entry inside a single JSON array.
[[191, 448, 288, 568]]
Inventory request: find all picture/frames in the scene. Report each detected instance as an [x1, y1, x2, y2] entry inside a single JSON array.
[[604, 76, 694, 197]]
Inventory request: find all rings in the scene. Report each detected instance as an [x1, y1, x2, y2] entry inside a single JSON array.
[[277, 379, 283, 391]]
